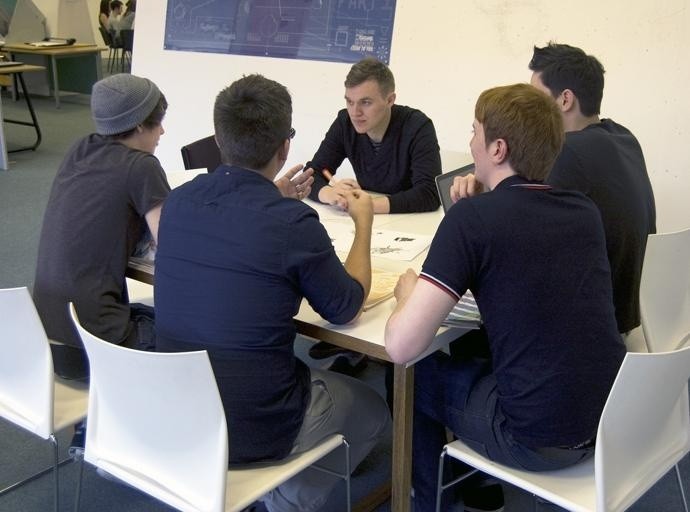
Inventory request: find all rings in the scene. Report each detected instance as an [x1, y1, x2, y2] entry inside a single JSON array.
[[295, 185, 303, 193]]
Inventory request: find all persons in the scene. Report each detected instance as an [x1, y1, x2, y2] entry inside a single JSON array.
[[117, 0, 135, 42], [98, 1, 109, 29], [380, 80, 627, 509], [31, 72, 172, 387], [299, 58, 443, 215], [105, 1, 124, 45], [532, 43, 657, 337], [151, 73, 394, 512]]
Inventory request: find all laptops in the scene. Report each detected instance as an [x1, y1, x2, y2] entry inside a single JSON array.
[[434, 163, 491, 216]]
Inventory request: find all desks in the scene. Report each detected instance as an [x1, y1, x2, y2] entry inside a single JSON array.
[[1, 40, 108, 109], [123, 190, 476, 512], [1, 61, 47, 158]]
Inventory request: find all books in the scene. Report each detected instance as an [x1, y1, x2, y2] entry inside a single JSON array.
[[440, 288, 481, 330], [364, 269, 399, 311]]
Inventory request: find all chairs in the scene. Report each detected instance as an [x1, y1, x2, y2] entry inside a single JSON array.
[[434, 347, 690, 512], [619, 231, 690, 352], [0, 286, 89, 511], [67, 302, 352, 510], [99, 27, 134, 75], [437, 149, 477, 179]]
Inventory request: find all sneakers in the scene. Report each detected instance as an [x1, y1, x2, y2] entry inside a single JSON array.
[[309, 341, 353, 360], [328, 354, 369, 377], [462, 483, 505, 512], [69, 434, 86, 457]]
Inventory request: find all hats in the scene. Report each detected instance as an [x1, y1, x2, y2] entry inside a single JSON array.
[[90, 73, 161, 136]]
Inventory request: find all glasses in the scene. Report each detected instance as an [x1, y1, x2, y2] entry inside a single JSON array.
[[287, 128, 295, 139]]
